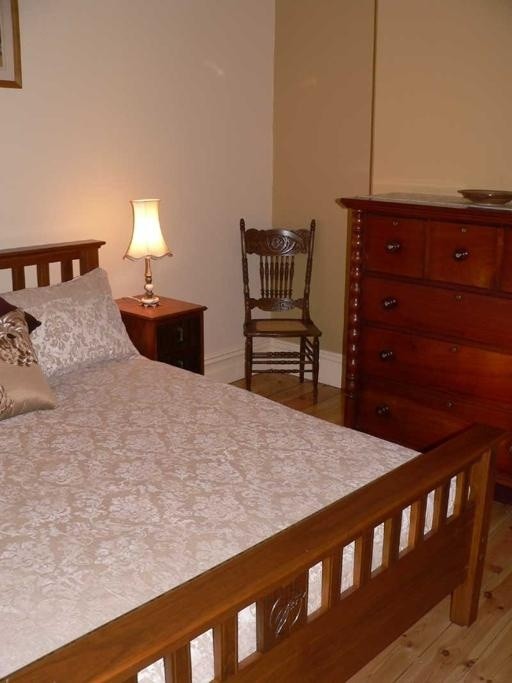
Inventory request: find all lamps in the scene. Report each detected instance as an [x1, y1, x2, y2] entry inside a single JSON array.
[[123, 197, 175, 309]]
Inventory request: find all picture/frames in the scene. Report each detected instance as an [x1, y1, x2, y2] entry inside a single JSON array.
[[1, 0, 23, 89]]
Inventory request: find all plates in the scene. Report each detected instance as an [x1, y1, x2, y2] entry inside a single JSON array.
[[458, 189, 511, 205]]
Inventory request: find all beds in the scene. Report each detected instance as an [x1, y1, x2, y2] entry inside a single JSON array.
[[0, 239, 512, 683]]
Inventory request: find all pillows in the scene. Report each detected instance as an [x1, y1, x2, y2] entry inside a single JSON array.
[[0, 265, 141, 421]]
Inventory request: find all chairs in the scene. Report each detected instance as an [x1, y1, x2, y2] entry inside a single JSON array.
[[239, 217, 323, 398]]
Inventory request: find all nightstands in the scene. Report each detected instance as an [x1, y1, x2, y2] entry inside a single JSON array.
[[112, 312, 209, 376]]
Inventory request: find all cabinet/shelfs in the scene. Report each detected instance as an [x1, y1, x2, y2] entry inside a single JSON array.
[[336, 207, 511, 507]]
